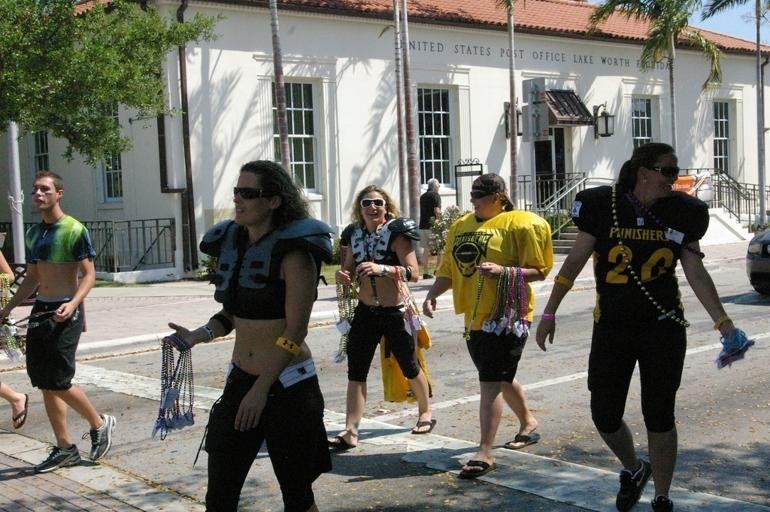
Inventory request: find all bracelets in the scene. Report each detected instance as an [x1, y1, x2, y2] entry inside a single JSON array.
[[378, 263, 412, 281], [210, 313, 233, 336], [202, 325, 214, 343], [276, 335, 301, 357], [713, 314, 732, 330], [541, 313, 555, 321], [553, 274, 573, 289]]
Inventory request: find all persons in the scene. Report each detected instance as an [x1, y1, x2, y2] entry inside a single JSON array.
[[163, 158, 341, 511], [420, 172, 556, 480], [325, 184, 437, 450], [419, 177, 443, 278], [535, 143, 755, 511], [0, 249, 30, 429], [0, 168, 118, 473]]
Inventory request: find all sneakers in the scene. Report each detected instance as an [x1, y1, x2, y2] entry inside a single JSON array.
[[90, 414, 116, 461], [34, 445, 80, 473], [616, 460, 673, 512]]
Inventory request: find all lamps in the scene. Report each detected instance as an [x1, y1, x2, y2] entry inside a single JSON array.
[[504, 96, 523, 138], [593, 100, 616, 139]]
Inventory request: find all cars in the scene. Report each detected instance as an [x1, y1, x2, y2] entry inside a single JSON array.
[[745, 210, 770, 295]]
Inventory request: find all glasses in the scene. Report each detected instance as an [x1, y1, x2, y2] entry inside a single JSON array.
[[361, 198, 383, 208], [653, 165, 681, 177], [233, 187, 262, 199], [471, 190, 496, 199]]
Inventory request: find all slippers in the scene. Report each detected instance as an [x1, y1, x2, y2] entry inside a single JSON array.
[[461, 459, 496, 477], [12, 394, 28, 428], [507, 434, 540, 449], [330, 436, 356, 449], [417, 418, 436, 433]]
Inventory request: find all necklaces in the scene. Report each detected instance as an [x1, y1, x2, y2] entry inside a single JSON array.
[[151, 332, 196, 440], [628, 188, 705, 258], [389, 265, 423, 331], [334, 268, 357, 363], [462, 262, 531, 343], [0, 273, 25, 363]]
